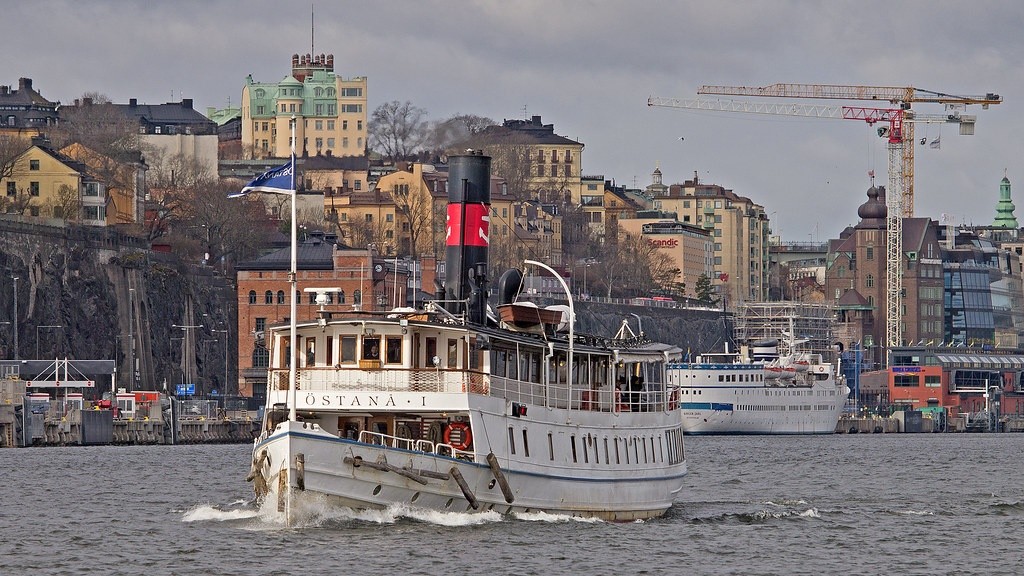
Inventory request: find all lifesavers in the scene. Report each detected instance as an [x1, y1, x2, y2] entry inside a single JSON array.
[[444, 422, 472, 451]]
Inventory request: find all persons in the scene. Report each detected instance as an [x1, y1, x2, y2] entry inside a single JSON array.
[[364, 344, 380, 360]]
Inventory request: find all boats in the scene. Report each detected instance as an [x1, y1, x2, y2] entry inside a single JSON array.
[[496, 301, 562, 336], [245, 115, 689, 529], [662, 310, 850, 438]]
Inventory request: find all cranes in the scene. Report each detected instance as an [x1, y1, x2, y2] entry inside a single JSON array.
[[697, 82, 1003, 219], [646, 92, 981, 366]]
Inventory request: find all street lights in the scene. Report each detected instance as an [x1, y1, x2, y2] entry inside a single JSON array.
[[211, 330, 228, 408], [172, 324, 204, 415], [13, 276, 21, 361], [36, 325, 62, 360], [129, 286, 148, 391]]
